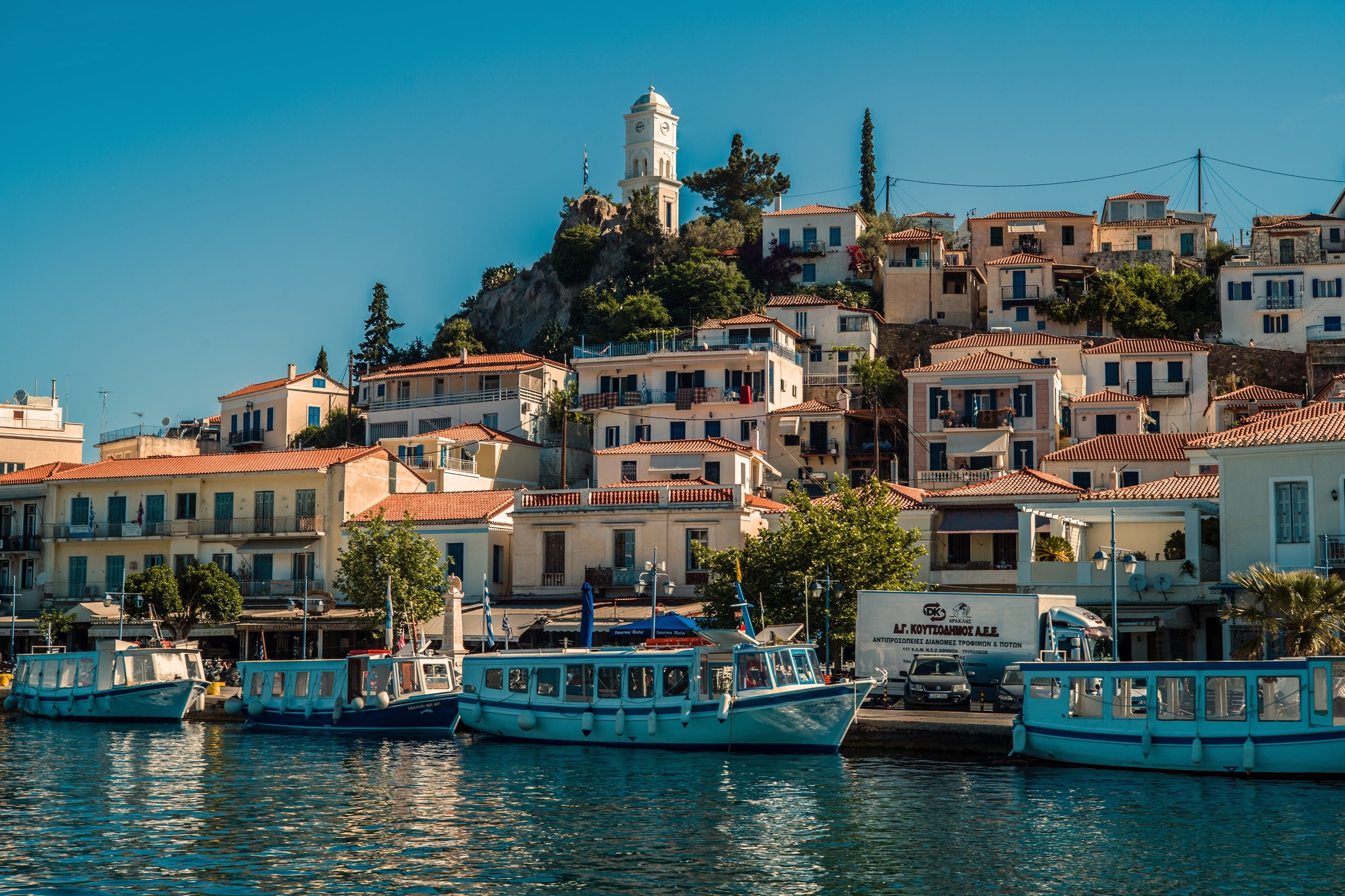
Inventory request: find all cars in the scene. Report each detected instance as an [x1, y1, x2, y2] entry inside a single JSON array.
[[990, 665, 1163, 714]]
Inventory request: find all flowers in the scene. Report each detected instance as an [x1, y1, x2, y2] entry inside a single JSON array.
[[9, 535, 20, 540]]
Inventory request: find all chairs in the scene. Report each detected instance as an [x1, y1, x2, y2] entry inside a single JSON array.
[[794, 241, 821, 252], [1328, 539, 1345, 563]]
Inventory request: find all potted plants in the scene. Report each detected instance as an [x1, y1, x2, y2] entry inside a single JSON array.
[[938, 410, 969, 427]]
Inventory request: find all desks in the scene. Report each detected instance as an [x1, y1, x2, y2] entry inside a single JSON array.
[[807, 243, 813, 252]]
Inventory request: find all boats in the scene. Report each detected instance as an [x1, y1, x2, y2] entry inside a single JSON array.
[[1007, 650, 1345, 783], [223, 598, 463, 738], [457, 581, 890, 757], [4, 603, 212, 726]]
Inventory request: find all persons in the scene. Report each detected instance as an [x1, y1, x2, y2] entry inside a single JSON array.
[[1155, 658, 1300, 718], [1095, 684, 1102, 695], [0, 653, 2, 664], [75, 642, 82, 652], [389, 664, 447, 695], [770, 631, 786, 646], [211, 657, 237, 671], [758, 672, 768, 687]]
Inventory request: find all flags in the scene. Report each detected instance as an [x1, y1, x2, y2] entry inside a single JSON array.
[[502, 614, 513, 641], [45, 629, 52, 652], [484, 577, 495, 648], [386, 578, 393, 648], [421, 628, 426, 649], [735, 557, 742, 582], [397, 628, 406, 649]]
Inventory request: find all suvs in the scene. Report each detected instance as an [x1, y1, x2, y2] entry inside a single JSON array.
[[899, 652, 975, 711]]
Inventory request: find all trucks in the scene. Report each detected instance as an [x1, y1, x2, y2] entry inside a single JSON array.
[[855, 588, 1121, 710]]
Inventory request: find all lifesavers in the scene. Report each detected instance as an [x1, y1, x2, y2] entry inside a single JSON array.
[[662, 666, 688, 694], [742, 660, 767, 687]]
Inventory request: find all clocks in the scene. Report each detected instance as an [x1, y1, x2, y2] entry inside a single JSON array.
[[634, 120, 670, 135]]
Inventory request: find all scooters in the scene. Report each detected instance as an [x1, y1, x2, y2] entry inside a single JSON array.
[[186, 656, 242, 688]]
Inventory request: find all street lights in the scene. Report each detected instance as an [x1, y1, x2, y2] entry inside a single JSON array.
[[810, 560, 846, 675], [1092, 506, 1139, 662], [101, 568, 144, 641], [285, 575, 325, 661], [633, 546, 676, 639]]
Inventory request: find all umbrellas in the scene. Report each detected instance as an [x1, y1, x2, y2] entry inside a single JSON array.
[[580, 583, 593, 648]]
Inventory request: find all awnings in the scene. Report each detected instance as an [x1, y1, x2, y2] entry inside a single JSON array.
[[1114, 611, 1194, 633], [544, 621, 633, 633], [404, 614, 541, 642], [608, 614, 703, 636]]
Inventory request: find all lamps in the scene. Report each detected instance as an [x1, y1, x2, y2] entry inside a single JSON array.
[[77, 492, 80, 499], [808, 222, 810, 226], [1031, 269, 1033, 273], [1001, 392, 1003, 397], [640, 410, 643, 425], [1127, 415, 1131, 419], [747, 361, 750, 371], [1006, 270, 1008, 274], [683, 364, 686, 369], [958, 393, 961, 398], [616, 369, 619, 374], [1331, 489, 1338, 501], [245, 401, 252, 412], [709, 411, 713, 418], [12, 511, 16, 517], [114, 491, 117, 496], [1082, 416, 1086, 421], [1010, 351, 1012, 357], [409, 440, 412, 446]]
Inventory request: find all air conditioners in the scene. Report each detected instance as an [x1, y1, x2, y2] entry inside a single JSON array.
[[863, 263, 873, 271], [1094, 487, 1107, 490], [83, 585, 98, 595], [757, 345, 768, 351], [523, 402, 531, 411]]
